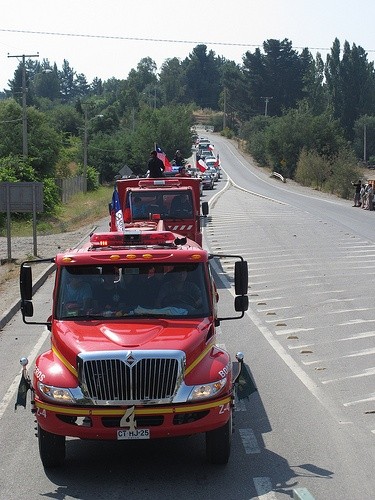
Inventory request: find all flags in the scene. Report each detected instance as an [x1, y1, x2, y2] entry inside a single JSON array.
[[111, 190, 124, 231], [14, 373, 29, 410], [208, 145, 214, 150], [214, 155, 220, 167], [233, 360, 257, 400], [196, 157, 208, 172], [155, 143, 172, 172]]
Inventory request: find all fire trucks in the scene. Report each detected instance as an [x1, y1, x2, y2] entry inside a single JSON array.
[[108, 177, 209, 249], [19, 228, 249, 467]]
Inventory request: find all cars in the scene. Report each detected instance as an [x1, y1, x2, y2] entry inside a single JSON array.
[[161, 137, 223, 189]]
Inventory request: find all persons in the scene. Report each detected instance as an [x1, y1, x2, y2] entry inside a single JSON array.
[[129, 195, 193, 218], [173, 149, 187, 165], [174, 166, 191, 177], [351, 180, 374, 210], [147, 150, 165, 177], [57, 267, 203, 311]]
